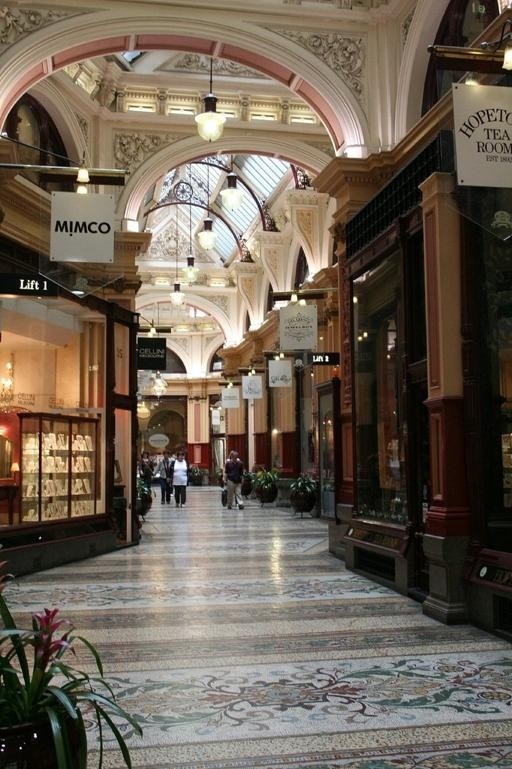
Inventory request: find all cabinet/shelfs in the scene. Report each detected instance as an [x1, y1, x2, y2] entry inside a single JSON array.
[[17, 412, 99, 526]]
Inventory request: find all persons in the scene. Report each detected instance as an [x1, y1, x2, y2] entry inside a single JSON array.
[[223, 450, 241, 506], [154, 450, 173, 505], [167, 451, 175, 496], [167, 452, 193, 508], [225, 452, 245, 509], [136, 453, 144, 478], [140, 451, 155, 489]]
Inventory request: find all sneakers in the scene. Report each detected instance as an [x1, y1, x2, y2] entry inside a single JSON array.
[[228, 505, 244, 510], [161, 493, 186, 508]]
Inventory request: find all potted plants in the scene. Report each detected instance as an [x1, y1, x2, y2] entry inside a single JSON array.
[[135, 475, 152, 515], [0, 543, 144, 769], [242, 464, 318, 512], [192, 466, 206, 486]]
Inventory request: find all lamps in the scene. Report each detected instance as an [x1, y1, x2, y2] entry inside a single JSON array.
[[1, 134, 92, 184], [196, 57, 226, 142], [169, 154, 243, 305]]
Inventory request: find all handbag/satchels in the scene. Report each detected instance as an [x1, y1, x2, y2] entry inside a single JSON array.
[[222, 490, 227, 507]]
